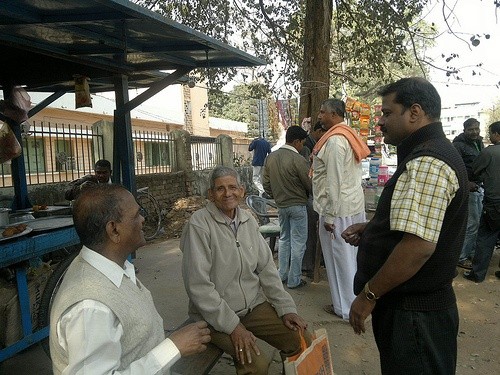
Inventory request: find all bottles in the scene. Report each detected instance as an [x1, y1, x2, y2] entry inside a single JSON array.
[[365, 157, 393, 209], [0, 209, 9, 227]]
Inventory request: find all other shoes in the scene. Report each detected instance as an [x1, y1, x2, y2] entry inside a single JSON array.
[[462, 271, 483, 283], [322, 304, 337, 315], [264, 192, 271, 199], [457, 259, 473, 270], [289, 279, 306, 289], [495, 271, 500, 278], [452, 268, 458, 280]]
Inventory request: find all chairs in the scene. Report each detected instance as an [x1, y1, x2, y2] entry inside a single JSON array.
[[246, 194, 281, 255]]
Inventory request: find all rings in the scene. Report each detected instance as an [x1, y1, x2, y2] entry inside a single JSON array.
[[238, 349, 243, 352]]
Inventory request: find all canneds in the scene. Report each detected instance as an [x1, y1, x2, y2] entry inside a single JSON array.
[[364, 186, 384, 209], [378, 166, 389, 185], [369, 158, 380, 178]]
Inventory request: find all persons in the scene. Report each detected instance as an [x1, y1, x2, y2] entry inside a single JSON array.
[[309, 99, 372, 318], [249, 131, 271, 198], [463, 121, 500, 283], [179, 165, 308, 375], [64, 160, 116, 200], [341, 77, 470, 375], [452, 118, 485, 269], [262, 110, 327, 289], [48, 183, 211, 375]]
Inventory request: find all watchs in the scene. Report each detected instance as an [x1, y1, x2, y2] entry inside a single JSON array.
[[362, 283, 380, 303]]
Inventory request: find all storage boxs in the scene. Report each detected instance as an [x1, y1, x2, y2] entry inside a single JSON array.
[[0, 267, 54, 347]]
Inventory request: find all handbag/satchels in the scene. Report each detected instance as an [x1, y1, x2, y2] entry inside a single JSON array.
[[284, 324, 333, 375], [72, 74, 92, 109], [0, 120, 22, 164]]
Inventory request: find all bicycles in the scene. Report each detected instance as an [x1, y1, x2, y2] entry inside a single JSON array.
[[135, 186, 162, 242]]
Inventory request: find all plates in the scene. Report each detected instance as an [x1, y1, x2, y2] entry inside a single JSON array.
[[0, 227, 33, 240]]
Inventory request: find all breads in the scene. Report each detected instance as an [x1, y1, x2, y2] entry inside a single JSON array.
[[33, 206, 48, 210], [2, 223, 28, 238]]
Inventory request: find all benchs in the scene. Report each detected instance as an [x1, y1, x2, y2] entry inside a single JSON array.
[[167, 316, 224, 375]]
